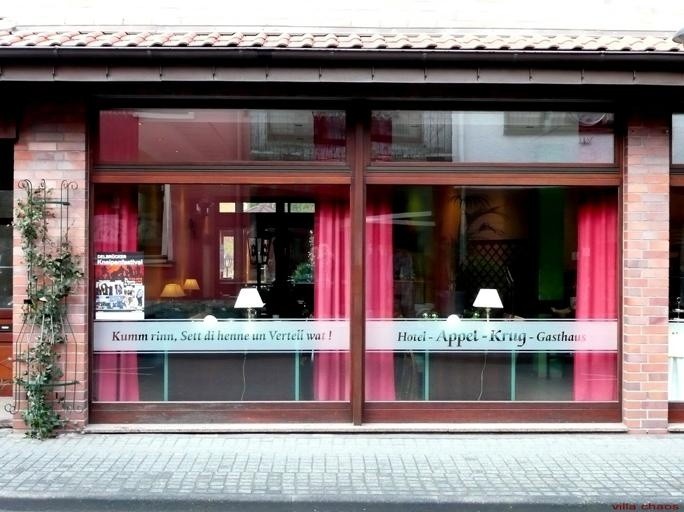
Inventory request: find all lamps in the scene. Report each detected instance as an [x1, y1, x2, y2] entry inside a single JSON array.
[[472, 288, 504, 321], [181, 279, 200, 296], [233, 287, 266, 319], [159, 281, 184, 302]]
